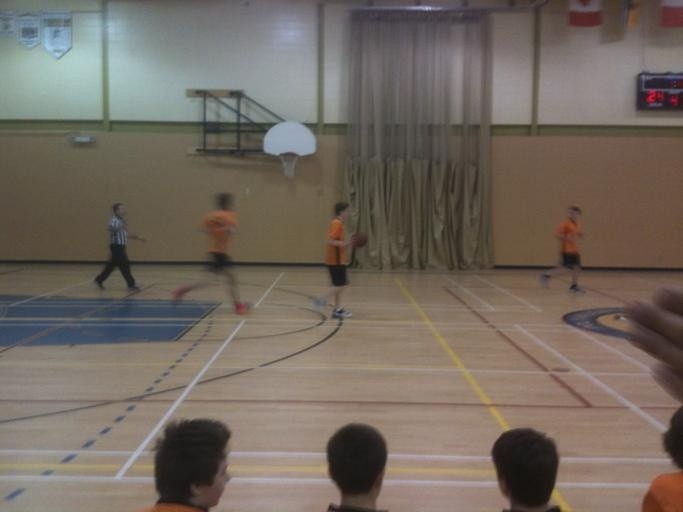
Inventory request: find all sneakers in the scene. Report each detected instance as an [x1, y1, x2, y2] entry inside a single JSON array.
[[540, 272, 585, 294], [94, 277, 139, 291], [330, 305, 353, 317]]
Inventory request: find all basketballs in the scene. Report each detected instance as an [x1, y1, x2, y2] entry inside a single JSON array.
[[351, 231, 368, 247]]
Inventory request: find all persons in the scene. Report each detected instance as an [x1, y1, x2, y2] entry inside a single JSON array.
[[640, 406, 683, 512], [490, 428, 562, 511], [617, 284, 682, 403], [538, 204, 585, 293], [324, 422, 390, 512], [94, 202, 145, 289], [126, 417, 234, 511], [169, 191, 250, 314], [306, 203, 366, 317]]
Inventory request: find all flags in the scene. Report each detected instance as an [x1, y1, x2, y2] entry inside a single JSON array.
[[659, 1, 682, 29], [566, 0, 604, 27], [620, 1, 642, 31]]
